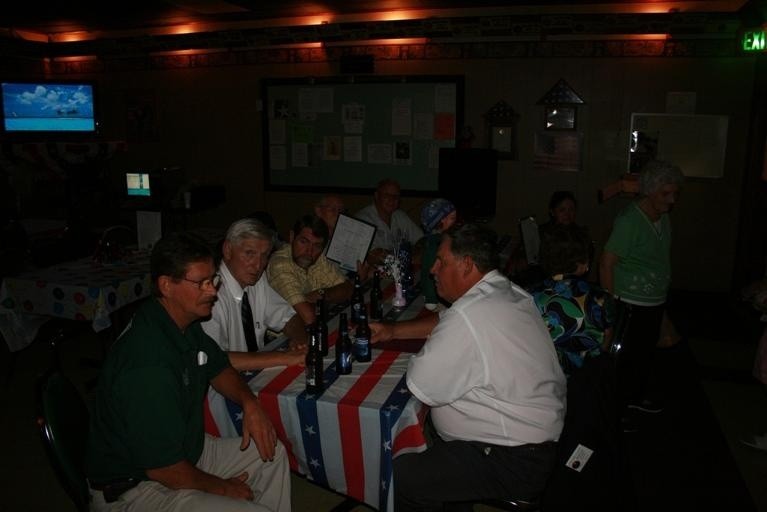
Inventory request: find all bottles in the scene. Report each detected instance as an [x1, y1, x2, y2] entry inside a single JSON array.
[[405, 241, 415, 287], [312, 298, 327, 355], [356, 304, 372, 361], [351, 274, 363, 323], [336, 313, 352, 375], [371, 271, 384, 318], [306, 324, 324, 395]]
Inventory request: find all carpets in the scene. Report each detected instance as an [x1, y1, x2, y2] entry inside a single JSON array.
[[524, 336, 758, 512]]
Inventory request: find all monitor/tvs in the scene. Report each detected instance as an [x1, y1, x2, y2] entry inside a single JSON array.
[[1, 79, 97, 134], [120, 167, 152, 199]]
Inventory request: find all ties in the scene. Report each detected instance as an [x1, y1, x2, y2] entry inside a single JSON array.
[[241, 291, 264, 376]]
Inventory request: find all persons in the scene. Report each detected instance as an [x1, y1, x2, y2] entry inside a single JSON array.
[[738, 328, 767, 452], [201, 218, 317, 370], [392, 223, 569, 512], [82, 230, 292, 512], [526, 225, 617, 377], [354, 178, 424, 251], [266, 214, 355, 328], [598, 162, 684, 434], [538, 191, 577, 241], [314, 194, 369, 282]]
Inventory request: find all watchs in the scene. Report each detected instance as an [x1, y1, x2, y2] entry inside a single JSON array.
[[316, 288, 327, 302]]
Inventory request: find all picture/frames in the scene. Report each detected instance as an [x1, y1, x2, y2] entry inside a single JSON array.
[[544, 106, 577, 130], [488, 125, 515, 160], [626, 112, 728, 179], [665, 92, 696, 113], [259, 74, 464, 198]]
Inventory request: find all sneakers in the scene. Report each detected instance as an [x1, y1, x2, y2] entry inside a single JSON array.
[[626, 397, 666, 414], [621, 418, 642, 433]]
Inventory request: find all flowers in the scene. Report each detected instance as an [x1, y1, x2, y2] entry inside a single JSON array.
[[381, 243, 411, 291]]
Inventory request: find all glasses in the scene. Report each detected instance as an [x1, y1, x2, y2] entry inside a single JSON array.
[[174, 275, 222, 292]]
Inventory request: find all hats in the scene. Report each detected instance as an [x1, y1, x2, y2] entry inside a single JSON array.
[[420, 197, 456, 233]]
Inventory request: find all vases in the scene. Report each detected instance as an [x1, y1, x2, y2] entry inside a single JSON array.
[[392, 283, 406, 306]]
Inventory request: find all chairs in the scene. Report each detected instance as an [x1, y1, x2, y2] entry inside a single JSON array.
[[98, 226, 137, 251], [33, 364, 91, 512], [0, 220, 27, 375], [449, 352, 598, 512]]
